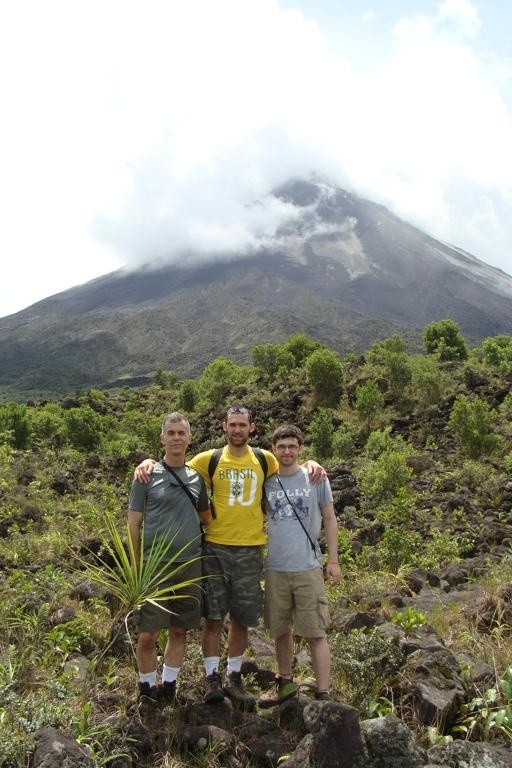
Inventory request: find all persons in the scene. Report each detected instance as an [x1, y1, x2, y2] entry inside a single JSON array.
[[124, 410, 216, 721], [132, 405, 328, 710], [256, 424, 345, 711]]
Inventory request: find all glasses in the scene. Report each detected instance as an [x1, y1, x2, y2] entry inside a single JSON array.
[[274, 444, 299, 451], [227, 407, 248, 415]]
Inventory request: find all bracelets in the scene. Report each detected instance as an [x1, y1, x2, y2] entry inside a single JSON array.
[[328, 559, 339, 565]]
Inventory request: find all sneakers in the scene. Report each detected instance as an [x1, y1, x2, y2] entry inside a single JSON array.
[[158, 680, 179, 710], [258, 676, 298, 708], [134, 681, 160, 719], [223, 671, 257, 708], [203, 672, 223, 704], [317, 690, 332, 700]]
